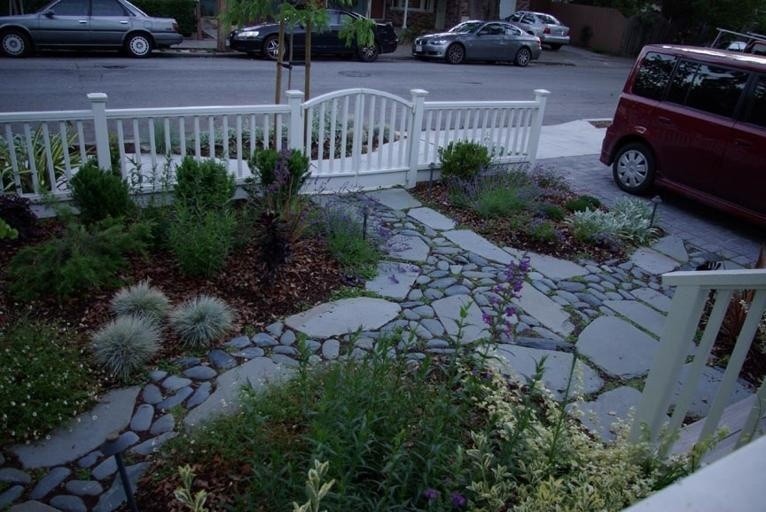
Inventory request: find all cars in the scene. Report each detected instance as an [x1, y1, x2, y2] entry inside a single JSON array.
[[225, 8, 399, 62], [718, 41, 749, 52], [0, 0, 183, 59], [743, 40, 766, 56], [410, 18, 541, 66], [501, 10, 571, 50]]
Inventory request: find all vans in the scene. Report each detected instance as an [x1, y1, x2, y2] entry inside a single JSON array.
[[598, 44, 765, 235]]
